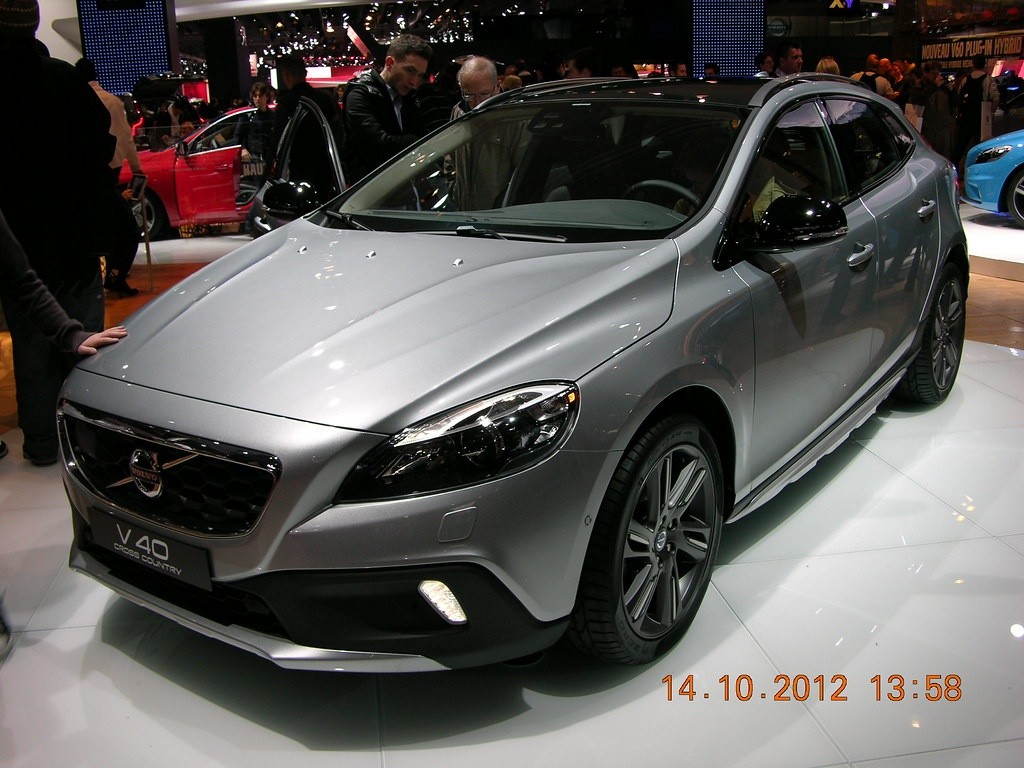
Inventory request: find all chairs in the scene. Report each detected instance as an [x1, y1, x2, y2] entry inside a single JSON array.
[[766, 128, 836, 195], [539, 124, 619, 202], [224, 114, 250, 145]]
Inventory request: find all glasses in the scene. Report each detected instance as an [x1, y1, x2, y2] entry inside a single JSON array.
[[461, 86, 495, 102]]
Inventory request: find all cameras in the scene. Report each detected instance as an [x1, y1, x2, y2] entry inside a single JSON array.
[[941, 72, 948, 84]]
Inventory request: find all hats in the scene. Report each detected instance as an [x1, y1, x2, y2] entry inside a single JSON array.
[[0, 0, 39, 33]]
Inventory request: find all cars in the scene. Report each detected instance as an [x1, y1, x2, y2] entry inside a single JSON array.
[[56, 68, 971, 680], [117, 103, 346, 245], [961, 126, 1024, 229]]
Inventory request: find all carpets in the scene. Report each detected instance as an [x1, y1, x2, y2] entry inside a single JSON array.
[[964, 272, 1024, 350], [103, 262, 214, 332]]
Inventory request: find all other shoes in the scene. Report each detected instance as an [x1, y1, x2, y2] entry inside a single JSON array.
[[105, 274, 138, 296], [21, 440, 58, 464], [0, 440, 8, 458]]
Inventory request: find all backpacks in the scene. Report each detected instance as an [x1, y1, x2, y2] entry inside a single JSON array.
[[859, 71, 880, 94], [960, 73, 988, 112]]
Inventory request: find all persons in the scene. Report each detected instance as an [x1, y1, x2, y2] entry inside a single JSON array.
[[0, 33, 718, 299], [749, 46, 1024, 204], [0, 0, 118, 468], [0, 215, 128, 658]]
